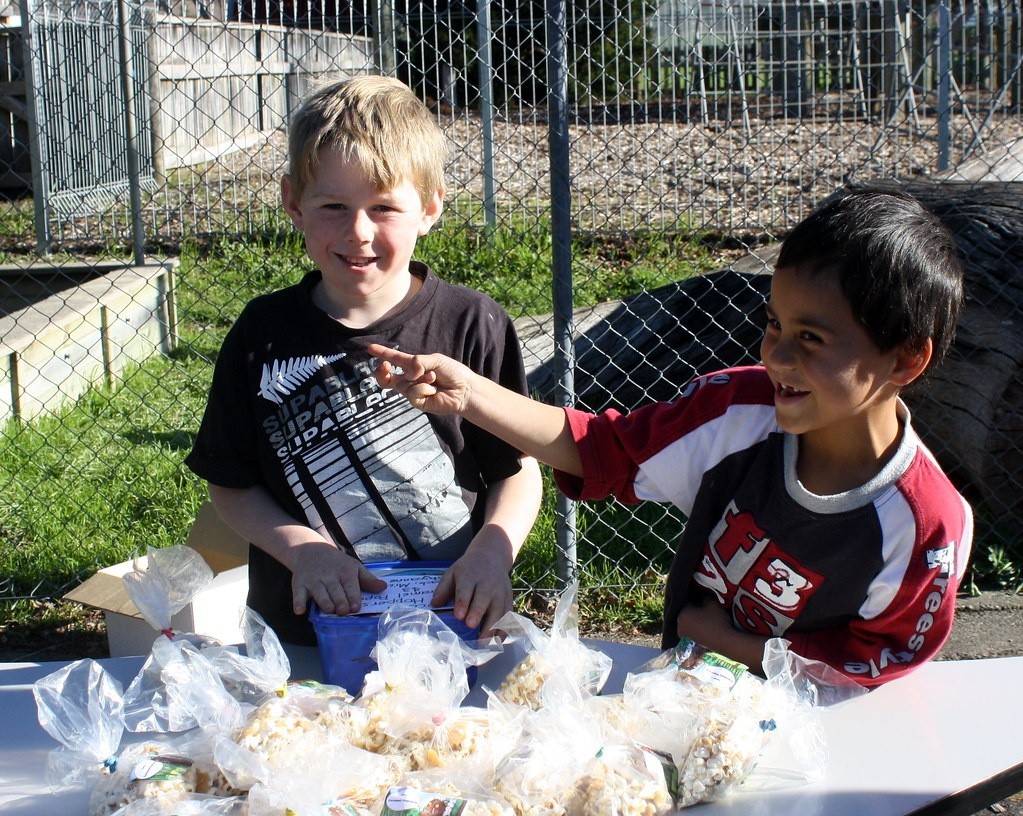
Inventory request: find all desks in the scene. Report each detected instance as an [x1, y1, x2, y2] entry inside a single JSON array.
[[1, 635, 1023, 816]]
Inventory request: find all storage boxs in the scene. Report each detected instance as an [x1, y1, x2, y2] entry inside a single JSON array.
[[62, 501, 250, 658], [310, 559, 477, 692]]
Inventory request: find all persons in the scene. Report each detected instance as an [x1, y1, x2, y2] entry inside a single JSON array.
[[367, 178, 974, 687], [184, 76, 542, 649]]
